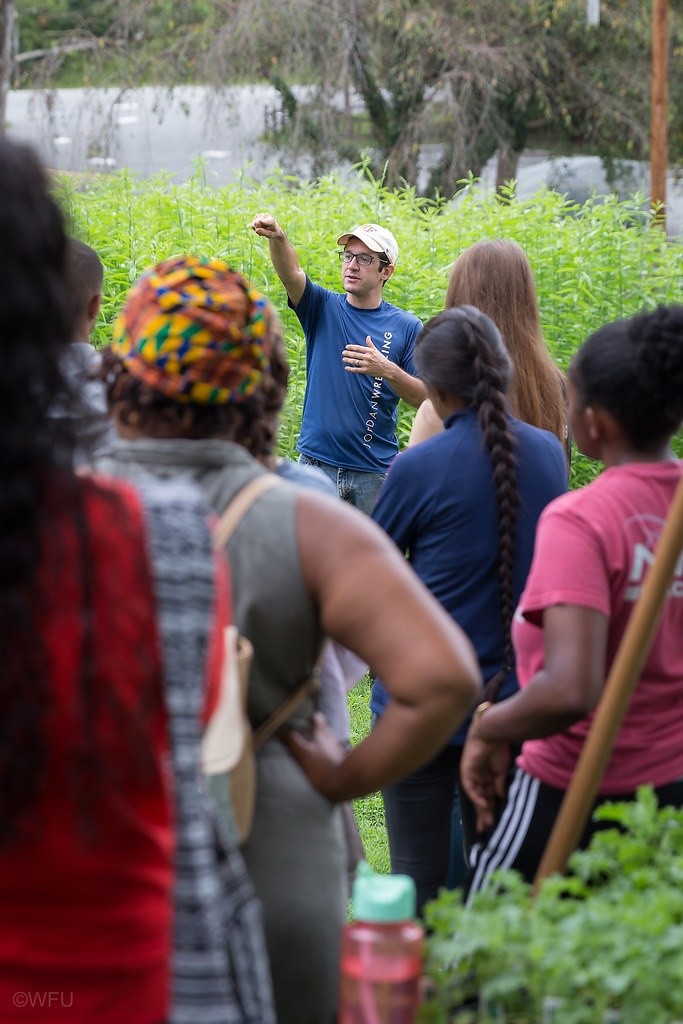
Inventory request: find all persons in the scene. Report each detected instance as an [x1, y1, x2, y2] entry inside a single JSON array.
[[0, 132, 174, 1024], [461, 303, 683, 905], [251, 211, 429, 514], [56, 236, 483, 1024], [368, 302, 570, 997], [410, 242, 574, 478]]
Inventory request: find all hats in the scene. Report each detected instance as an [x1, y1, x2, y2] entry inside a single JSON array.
[[336, 224, 399, 265], [112, 252, 278, 404]]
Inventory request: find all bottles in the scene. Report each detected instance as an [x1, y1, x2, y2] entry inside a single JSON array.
[[338, 861, 423, 1024]]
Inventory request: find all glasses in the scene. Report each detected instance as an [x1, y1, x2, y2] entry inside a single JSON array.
[[338, 251, 388, 266]]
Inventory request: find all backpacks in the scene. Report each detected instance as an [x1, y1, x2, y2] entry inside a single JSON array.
[[201, 474, 326, 847]]
